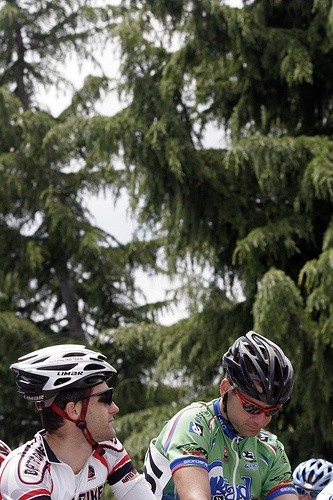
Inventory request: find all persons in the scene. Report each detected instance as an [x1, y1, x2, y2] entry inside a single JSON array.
[[293, 458, 333, 500], [143, 331, 298, 500], [0, 344, 157, 500]]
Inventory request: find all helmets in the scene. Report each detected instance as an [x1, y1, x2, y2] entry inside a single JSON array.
[[292, 457, 332, 491], [10, 344, 117, 403], [223, 330, 294, 405]]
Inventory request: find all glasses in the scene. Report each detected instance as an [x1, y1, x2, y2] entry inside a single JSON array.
[[75, 388, 114, 405], [235, 389, 282, 417], [295, 484, 321, 500]]
